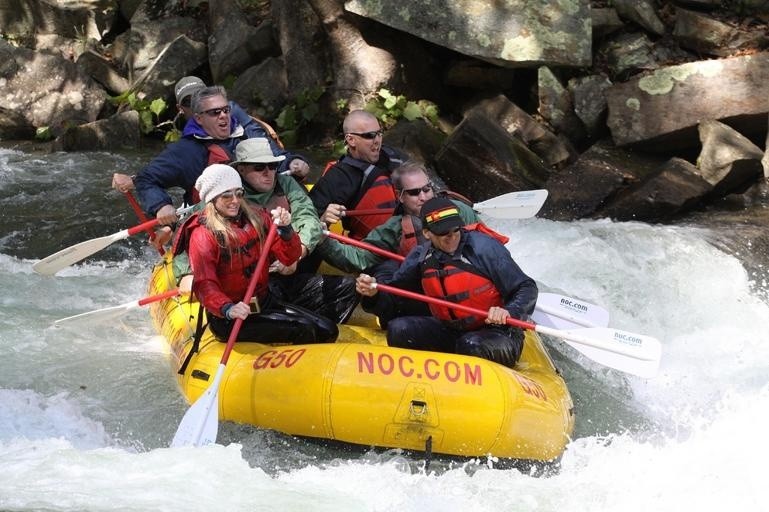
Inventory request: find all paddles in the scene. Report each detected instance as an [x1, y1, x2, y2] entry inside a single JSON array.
[[168, 210, 281, 447], [370, 282, 661, 378], [340, 189, 548, 219], [54, 265, 278, 327], [32, 170, 291, 275], [321, 229, 608, 327]]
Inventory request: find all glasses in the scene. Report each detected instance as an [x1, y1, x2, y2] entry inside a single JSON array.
[[221, 188, 245, 199], [434, 227, 460, 236], [182, 99, 191, 106], [350, 129, 384, 138], [254, 163, 277, 171], [404, 182, 431, 195]]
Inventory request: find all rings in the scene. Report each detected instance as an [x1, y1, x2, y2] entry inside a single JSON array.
[[241, 310, 246, 316], [336, 210, 342, 215]]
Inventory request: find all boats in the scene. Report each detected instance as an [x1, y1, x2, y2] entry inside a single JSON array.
[[144, 183, 578, 462]]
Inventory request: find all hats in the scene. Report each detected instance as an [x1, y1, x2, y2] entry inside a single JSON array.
[[195, 164, 245, 203], [175, 76, 207, 106], [199, 105, 231, 116], [419, 198, 464, 234], [229, 137, 286, 166]]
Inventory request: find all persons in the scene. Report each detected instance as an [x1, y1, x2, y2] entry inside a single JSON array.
[[134, 85, 311, 227], [316, 160, 489, 313], [354, 194, 540, 369], [111, 73, 280, 251], [171, 136, 361, 323], [187, 162, 340, 345], [306, 109, 402, 244]]
